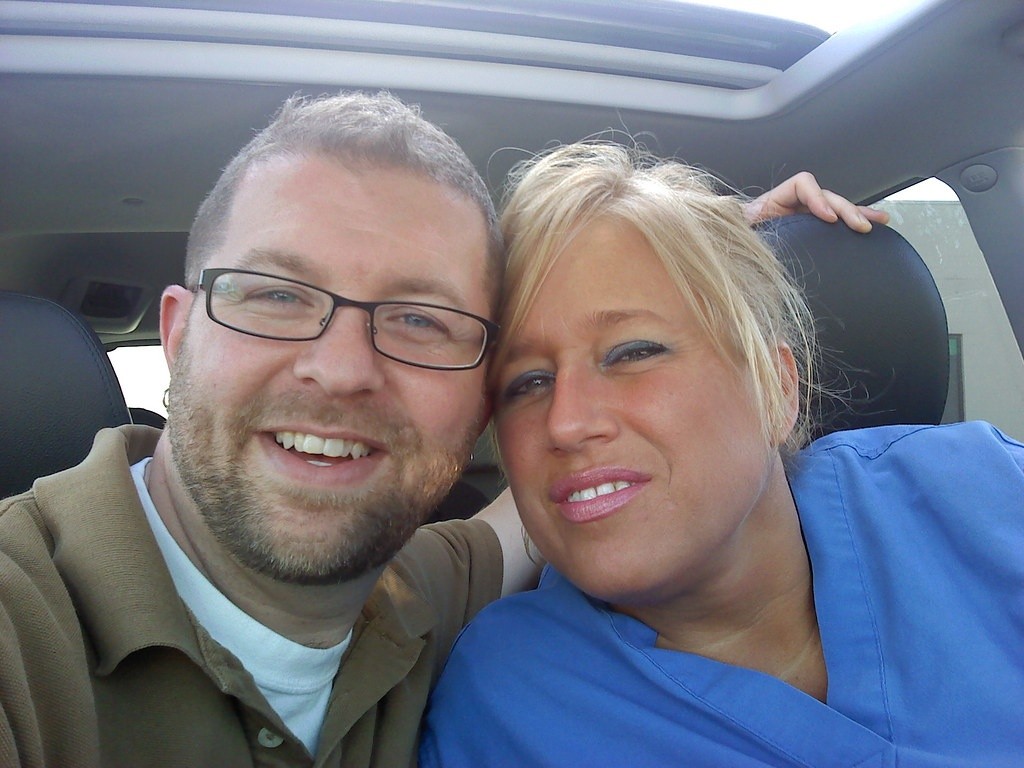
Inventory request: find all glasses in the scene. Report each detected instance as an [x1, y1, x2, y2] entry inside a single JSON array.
[[189, 266, 502, 370]]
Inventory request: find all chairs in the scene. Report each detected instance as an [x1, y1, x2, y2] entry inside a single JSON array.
[[754, 214, 950, 450], [0, 289, 134, 502]]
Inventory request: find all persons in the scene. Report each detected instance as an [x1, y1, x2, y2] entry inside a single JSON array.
[[0, 91, 891, 765], [425, 141, 1022, 768]]
